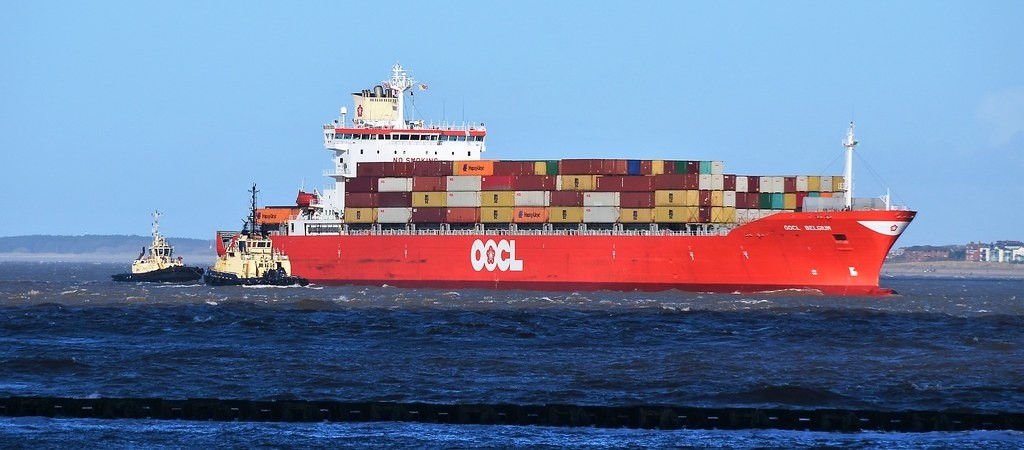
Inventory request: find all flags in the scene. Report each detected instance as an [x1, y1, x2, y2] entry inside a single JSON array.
[[216, 232, 227, 256], [419, 84, 428, 91], [229, 236, 240, 251]]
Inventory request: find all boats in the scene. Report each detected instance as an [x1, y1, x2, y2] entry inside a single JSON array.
[[110, 211, 204, 282], [202, 227, 310, 287]]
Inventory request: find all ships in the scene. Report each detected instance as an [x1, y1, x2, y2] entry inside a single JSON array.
[[610, 231, 613, 233], [216, 62, 917, 297]]
